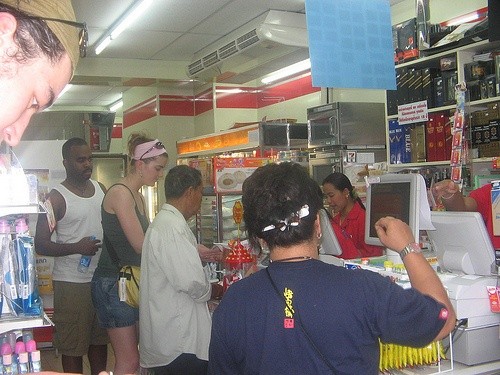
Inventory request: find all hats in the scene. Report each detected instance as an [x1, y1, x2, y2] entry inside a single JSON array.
[[0, 0, 81, 84]]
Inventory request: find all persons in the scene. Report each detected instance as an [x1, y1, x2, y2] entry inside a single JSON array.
[[0, 0, 80, 145], [322, 172, 384, 259], [35, 138, 106, 375], [434, 179, 500, 250], [138, 164, 224, 375], [209, 160, 456, 375], [90, 132, 218, 375]]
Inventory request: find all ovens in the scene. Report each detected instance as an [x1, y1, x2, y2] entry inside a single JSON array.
[[306, 103, 386, 148]]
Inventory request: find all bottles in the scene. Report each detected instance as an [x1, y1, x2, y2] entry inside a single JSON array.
[[0, 328, 41, 375], [77, 236, 97, 274]]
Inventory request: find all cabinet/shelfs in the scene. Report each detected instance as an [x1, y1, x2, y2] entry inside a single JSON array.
[[0, 144, 56, 334], [384, 38, 500, 168]]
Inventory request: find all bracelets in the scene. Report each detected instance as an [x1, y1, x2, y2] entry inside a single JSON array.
[[441, 193, 454, 199]]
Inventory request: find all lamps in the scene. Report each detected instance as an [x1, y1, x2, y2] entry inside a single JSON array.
[[108, 97, 123, 112], [93, 0, 153, 55]]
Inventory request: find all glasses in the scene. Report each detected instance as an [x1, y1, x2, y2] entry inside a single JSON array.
[[140, 141, 166, 160], [14, 16, 88, 58]]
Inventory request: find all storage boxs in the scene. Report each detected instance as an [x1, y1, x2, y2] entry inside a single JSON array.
[[387, 56, 500, 164]]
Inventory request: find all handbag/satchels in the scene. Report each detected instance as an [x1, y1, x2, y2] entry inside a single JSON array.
[[118, 265, 141, 310]]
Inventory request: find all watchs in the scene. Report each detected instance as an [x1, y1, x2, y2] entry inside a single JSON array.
[[400, 242, 422, 260]]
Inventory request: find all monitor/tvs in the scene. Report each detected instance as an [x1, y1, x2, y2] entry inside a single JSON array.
[[427, 211, 496, 276], [364, 173, 420, 264], [316, 209, 342, 256]]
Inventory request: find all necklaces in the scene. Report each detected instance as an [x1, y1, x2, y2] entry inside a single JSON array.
[[277, 256, 312, 261]]
